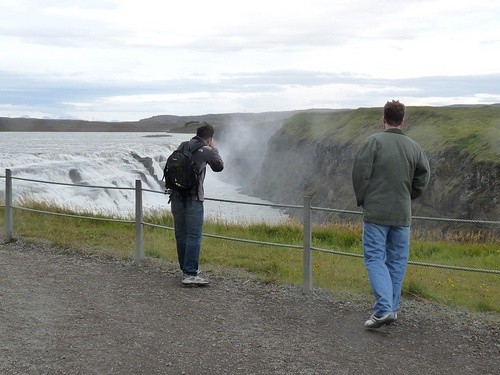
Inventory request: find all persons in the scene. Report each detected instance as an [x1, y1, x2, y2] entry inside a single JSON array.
[[162, 125, 223, 285], [352, 100, 430, 327]]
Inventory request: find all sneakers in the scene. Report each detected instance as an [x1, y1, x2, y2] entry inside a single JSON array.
[[386, 312, 397, 324], [180, 270, 202, 278], [364, 312, 394, 328], [182, 273, 210, 285]]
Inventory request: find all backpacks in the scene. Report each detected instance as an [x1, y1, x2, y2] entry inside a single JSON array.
[[162, 139, 209, 204]]
